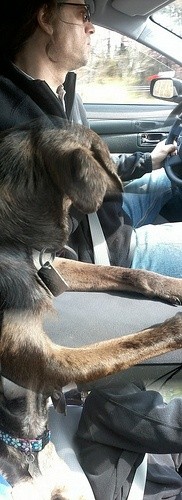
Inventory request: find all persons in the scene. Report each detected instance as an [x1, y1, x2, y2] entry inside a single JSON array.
[[74, 376, 182, 500], [0, 0, 182, 279]]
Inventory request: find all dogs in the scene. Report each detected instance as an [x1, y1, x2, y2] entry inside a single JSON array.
[[0, 114, 182, 500]]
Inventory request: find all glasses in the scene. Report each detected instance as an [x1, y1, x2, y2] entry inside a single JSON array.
[[47, 0, 96, 23]]
